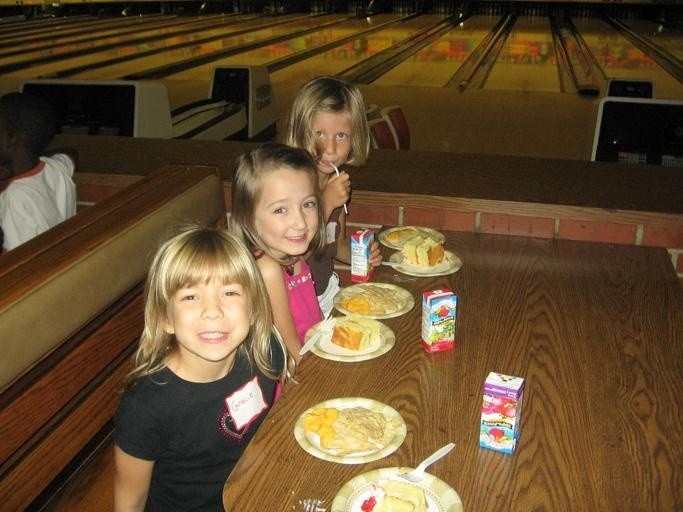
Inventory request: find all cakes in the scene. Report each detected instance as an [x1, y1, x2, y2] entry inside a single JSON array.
[[361, 479, 428, 512], [332, 316, 379, 352], [400, 236, 444, 269]]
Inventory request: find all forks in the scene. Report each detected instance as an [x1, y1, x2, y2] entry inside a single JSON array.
[[293, 314, 337, 356]]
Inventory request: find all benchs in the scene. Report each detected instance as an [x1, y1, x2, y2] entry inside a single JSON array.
[[1, 171, 228, 512]]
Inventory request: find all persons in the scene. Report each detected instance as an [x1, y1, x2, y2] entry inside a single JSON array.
[[0, 88, 79, 267], [224, 142, 328, 408], [282, 71, 381, 318], [108, 221, 292, 511]]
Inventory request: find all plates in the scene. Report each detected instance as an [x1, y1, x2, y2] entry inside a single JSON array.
[[330, 467, 464, 512], [378, 226, 446, 251], [389, 250, 463, 279], [293, 397, 407, 465], [304, 316, 396, 363], [333, 281, 416, 320]]
